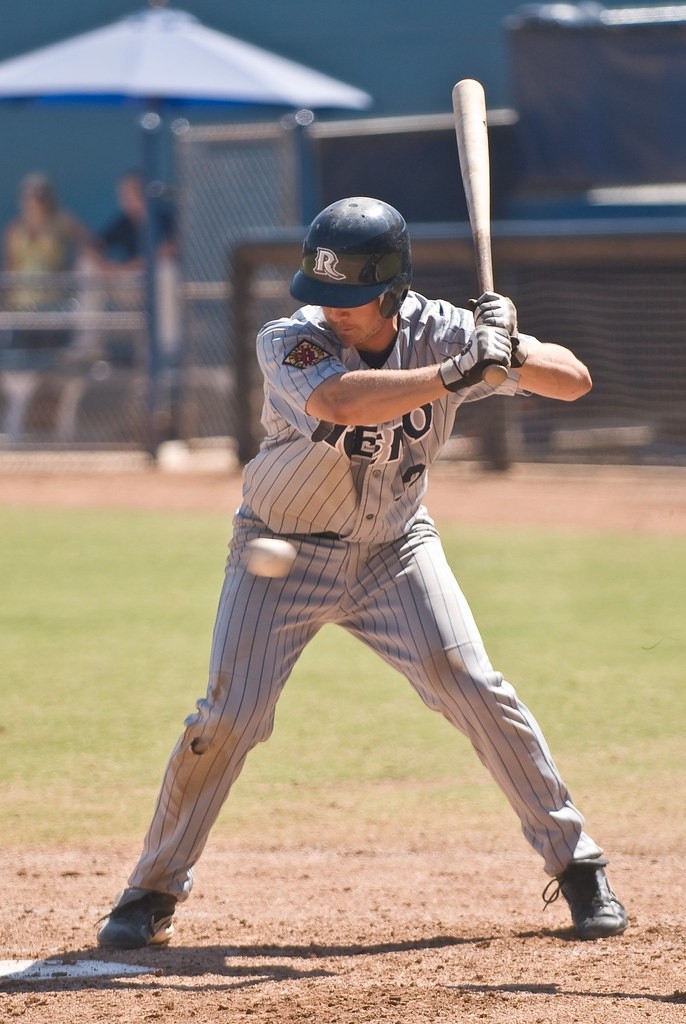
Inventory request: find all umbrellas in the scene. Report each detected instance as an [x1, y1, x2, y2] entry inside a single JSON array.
[[1, 0, 376, 111]]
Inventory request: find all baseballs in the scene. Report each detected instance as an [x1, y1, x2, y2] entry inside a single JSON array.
[[244, 537, 297, 578]]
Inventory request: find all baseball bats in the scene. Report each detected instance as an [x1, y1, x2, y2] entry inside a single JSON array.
[[452, 80, 510, 387]]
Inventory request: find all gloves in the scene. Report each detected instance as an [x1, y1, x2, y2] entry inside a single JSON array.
[[466, 291, 528, 368], [439, 325, 512, 393]]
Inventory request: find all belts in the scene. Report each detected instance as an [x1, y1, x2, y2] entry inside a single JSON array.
[[311, 531, 348, 540]]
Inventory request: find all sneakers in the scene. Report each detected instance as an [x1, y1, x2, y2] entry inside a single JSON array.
[[542, 859, 628, 939], [94, 888, 177, 950]]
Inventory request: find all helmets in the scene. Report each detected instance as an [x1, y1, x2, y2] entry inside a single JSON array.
[[290, 197, 413, 319]]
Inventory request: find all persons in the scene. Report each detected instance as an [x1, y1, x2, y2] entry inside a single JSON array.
[[97, 196, 626, 949], [0, 171, 180, 445]]
